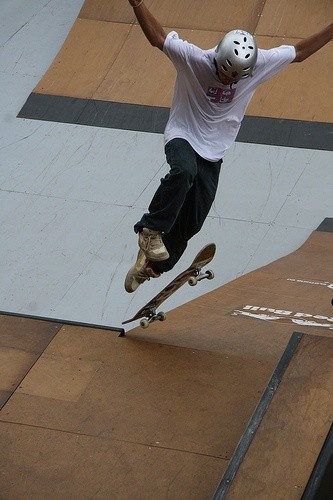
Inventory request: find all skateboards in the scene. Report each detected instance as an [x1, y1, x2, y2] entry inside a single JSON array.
[[121, 243, 216, 329]]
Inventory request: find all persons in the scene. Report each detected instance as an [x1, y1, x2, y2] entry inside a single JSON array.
[[123, 1, 333, 293]]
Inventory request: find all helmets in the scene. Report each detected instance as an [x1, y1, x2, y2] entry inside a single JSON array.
[[215, 30, 258, 80]]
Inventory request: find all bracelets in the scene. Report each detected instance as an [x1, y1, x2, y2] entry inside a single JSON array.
[[129, 0, 145, 8]]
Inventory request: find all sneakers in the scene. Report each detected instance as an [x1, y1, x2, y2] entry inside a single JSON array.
[[138, 228, 169, 261], [125, 249, 161, 293]]
